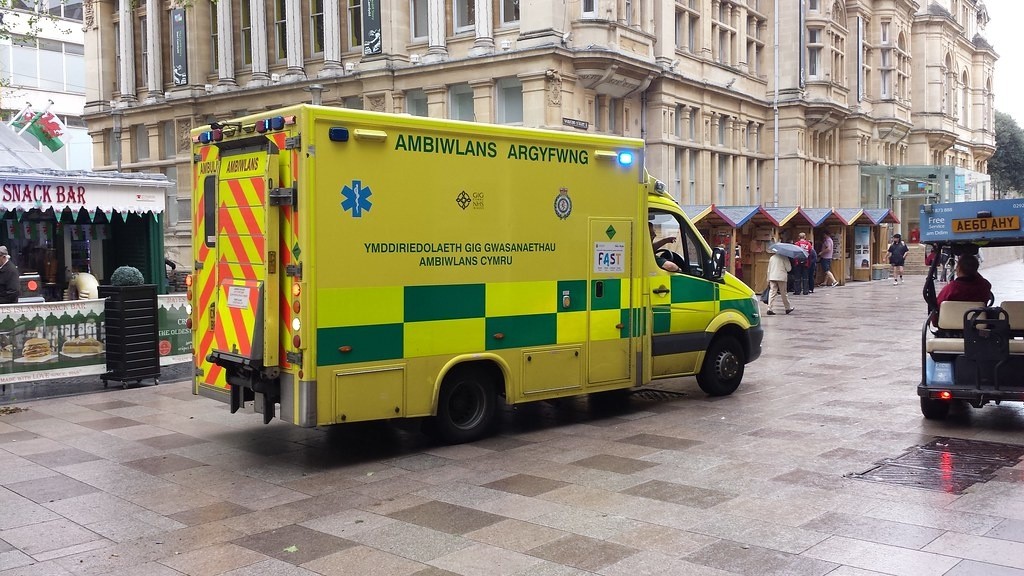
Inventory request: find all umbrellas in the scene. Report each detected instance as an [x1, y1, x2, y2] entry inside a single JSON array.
[[768, 243, 809, 260]]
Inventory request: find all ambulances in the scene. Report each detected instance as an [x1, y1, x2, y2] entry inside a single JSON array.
[[186, 103, 764, 446]]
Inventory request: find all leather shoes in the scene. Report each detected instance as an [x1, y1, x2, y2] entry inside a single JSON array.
[[786, 308, 794, 314], [767, 310, 775, 315]]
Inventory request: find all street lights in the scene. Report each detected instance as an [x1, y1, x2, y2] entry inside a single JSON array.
[[303, 84, 330, 107]]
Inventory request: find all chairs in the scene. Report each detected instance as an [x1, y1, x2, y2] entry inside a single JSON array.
[[926, 300, 987, 354], [998, 301, 1024, 355]]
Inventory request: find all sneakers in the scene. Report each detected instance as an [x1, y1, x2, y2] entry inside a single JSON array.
[[831, 282, 839, 288], [817, 283, 827, 288]]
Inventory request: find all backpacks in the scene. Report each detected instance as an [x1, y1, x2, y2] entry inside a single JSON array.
[[798, 253, 806, 266]]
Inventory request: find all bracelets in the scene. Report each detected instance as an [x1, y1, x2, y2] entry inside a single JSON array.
[[676, 267, 680, 271]]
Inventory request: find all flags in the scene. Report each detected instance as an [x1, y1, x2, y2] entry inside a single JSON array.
[[12, 107, 73, 153]]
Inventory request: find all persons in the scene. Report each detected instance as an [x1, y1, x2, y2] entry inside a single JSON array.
[[164, 257, 176, 286], [931, 255, 992, 337], [793, 232, 812, 295], [926, 242, 940, 280], [68, 263, 100, 300], [0, 245, 21, 304], [940, 243, 952, 281], [819, 230, 839, 288], [648, 222, 683, 272], [883, 234, 910, 285], [767, 254, 794, 315], [807, 240, 818, 293]]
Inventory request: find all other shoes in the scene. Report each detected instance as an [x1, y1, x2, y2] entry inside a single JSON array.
[[943, 279, 946, 281], [894, 282, 898, 284], [940, 278, 943, 282], [793, 293, 800, 295], [901, 280, 904, 283]]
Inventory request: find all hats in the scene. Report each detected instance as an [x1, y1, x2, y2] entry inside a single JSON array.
[[0, 246, 8, 255]]
